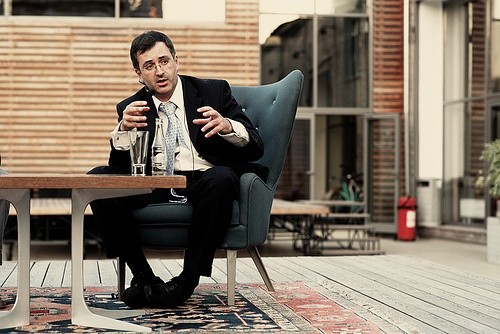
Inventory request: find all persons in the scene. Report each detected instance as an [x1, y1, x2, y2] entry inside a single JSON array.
[[84, 30, 266, 309]]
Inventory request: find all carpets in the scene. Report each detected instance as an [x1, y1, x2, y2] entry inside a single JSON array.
[[0, 280, 422, 334]]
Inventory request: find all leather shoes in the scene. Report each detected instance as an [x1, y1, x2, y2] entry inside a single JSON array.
[[121, 277, 156, 308], [144, 276, 193, 307]]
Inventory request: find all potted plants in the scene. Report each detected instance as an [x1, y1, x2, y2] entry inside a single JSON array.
[[476, 140, 500, 266]]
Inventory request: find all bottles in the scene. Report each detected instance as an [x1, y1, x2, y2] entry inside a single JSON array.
[[152, 118, 167, 176]]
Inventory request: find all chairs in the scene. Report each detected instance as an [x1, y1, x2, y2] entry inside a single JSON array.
[[117, 70, 303, 308]]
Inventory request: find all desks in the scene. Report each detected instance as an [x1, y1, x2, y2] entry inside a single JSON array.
[[0, 175, 187, 334]]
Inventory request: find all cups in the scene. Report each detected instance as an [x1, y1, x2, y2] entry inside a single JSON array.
[[128, 131, 149, 176]]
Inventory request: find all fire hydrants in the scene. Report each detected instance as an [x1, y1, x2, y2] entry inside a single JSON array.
[[398, 194, 418, 244]]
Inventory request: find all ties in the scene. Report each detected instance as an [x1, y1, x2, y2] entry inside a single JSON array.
[[158, 103, 187, 204]]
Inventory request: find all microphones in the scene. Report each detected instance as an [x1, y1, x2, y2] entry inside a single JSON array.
[[145, 85, 156, 95]]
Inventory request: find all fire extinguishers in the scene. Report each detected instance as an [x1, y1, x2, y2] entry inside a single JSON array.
[[397, 196, 417, 240]]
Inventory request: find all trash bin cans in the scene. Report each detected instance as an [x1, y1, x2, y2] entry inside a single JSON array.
[[416, 177, 443, 227]]
